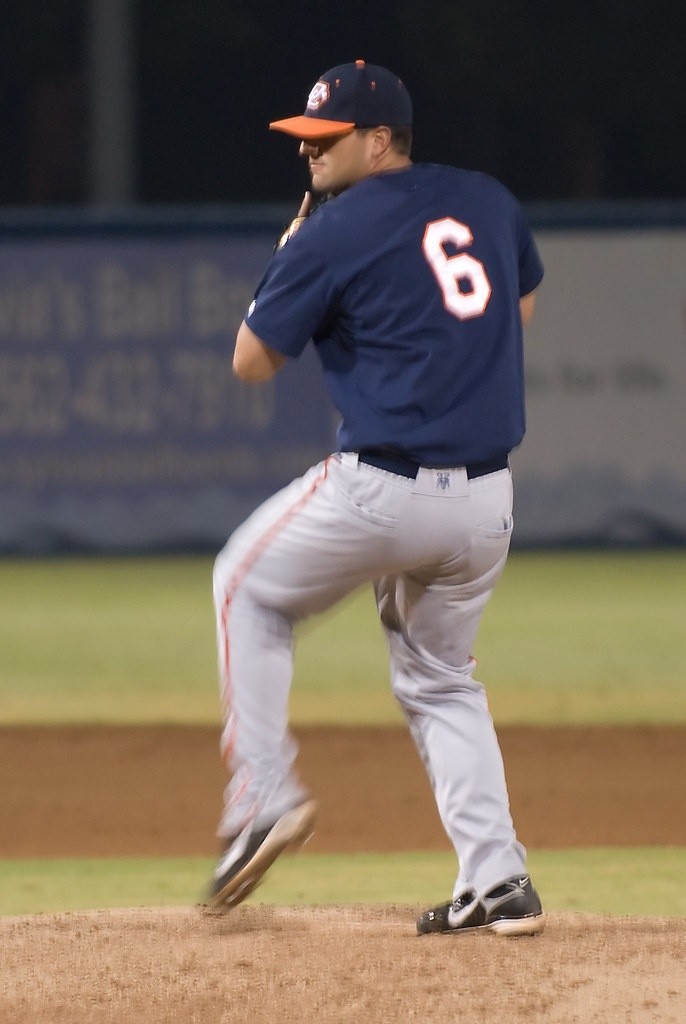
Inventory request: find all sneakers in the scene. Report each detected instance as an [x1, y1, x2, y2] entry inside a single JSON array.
[[197, 798, 319, 918], [417, 874, 545, 936]]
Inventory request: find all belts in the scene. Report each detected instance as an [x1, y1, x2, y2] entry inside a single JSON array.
[[360, 443, 509, 479]]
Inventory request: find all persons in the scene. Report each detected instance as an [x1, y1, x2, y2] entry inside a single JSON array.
[[199, 59, 546, 938]]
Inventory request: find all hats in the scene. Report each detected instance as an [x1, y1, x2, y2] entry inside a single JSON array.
[[268, 61, 414, 140]]
